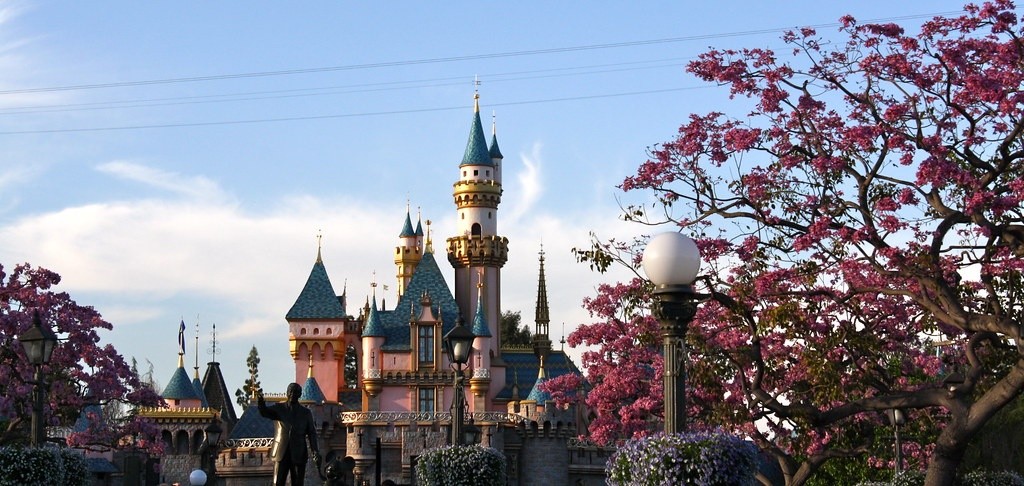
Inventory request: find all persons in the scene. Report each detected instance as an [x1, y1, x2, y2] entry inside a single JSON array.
[[313, 455, 346, 486], [257, 383, 323, 486]]
